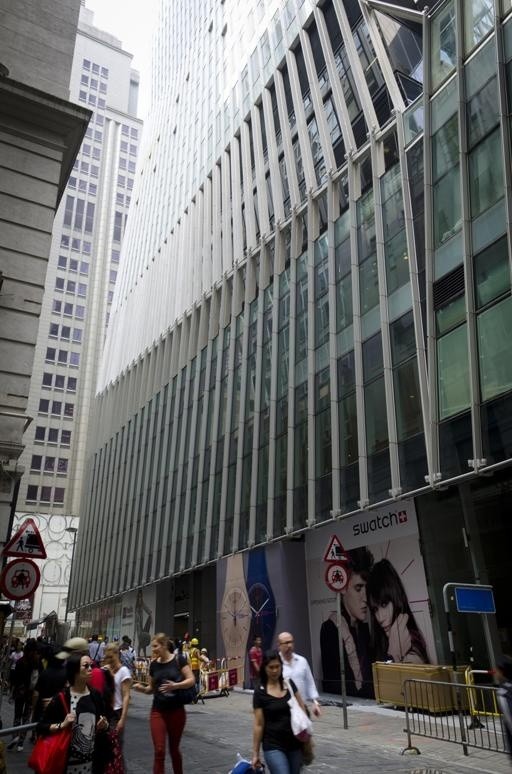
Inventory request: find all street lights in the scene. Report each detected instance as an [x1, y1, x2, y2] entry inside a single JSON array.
[[64, 526, 78, 622]]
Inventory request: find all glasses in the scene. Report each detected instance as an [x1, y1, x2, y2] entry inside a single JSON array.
[[81, 663, 94, 669]]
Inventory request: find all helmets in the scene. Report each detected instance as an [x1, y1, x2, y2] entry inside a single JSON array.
[[202, 648, 207, 653], [192, 638, 198, 644]]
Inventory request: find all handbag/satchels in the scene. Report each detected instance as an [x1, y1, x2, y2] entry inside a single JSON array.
[[287, 696, 313, 742], [176, 674, 196, 705], [28, 724, 72, 774]]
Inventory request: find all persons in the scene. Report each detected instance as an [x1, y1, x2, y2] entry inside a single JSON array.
[[335, 557, 427, 695], [0, 630, 212, 774], [250, 648, 308, 774], [248, 636, 263, 691], [277, 631, 322, 719], [319, 546, 412, 700]]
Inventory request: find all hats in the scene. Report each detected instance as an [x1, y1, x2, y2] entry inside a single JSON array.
[[55, 638, 89, 659]]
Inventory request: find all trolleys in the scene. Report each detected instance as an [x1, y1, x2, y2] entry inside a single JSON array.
[[201, 656, 241, 697]]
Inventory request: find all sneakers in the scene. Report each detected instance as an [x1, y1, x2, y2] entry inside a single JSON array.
[[17, 746, 23, 751], [7, 736, 20, 749]]
